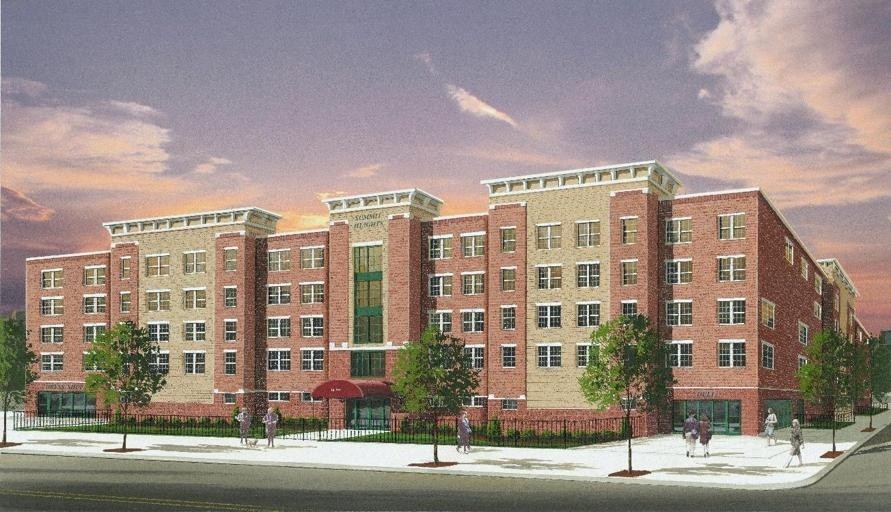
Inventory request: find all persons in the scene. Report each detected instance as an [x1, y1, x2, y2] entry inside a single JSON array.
[[783, 418, 804, 468], [682, 410, 698, 458], [698, 413, 712, 458], [763, 407, 778, 447], [260, 407, 278, 448], [462, 411, 472, 450], [237, 407, 251, 447], [455, 411, 468, 455]]
